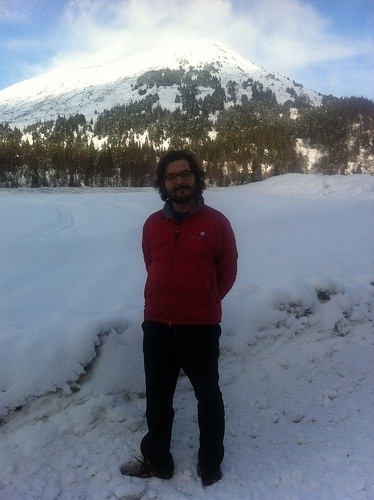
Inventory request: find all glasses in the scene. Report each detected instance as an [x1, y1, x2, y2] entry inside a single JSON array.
[[165, 170, 192, 181]]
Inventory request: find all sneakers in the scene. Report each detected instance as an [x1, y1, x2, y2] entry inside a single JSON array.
[[120, 457, 173, 479], [201, 471, 221, 486]]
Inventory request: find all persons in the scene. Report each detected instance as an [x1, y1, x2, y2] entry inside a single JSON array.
[[120, 150, 239, 487]]
